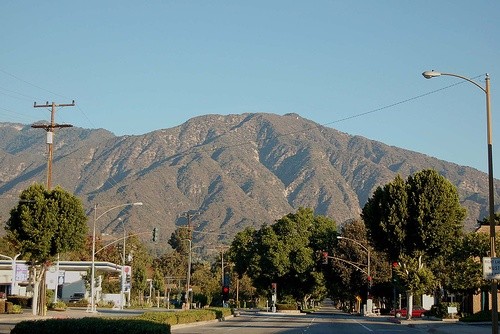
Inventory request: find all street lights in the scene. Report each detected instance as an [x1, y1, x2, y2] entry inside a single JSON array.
[[90, 201, 144, 313], [337, 236, 371, 298], [421, 68, 499, 334]]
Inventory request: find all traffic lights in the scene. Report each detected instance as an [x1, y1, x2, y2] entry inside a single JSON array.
[[322, 251, 329, 265], [152, 227, 157, 242]]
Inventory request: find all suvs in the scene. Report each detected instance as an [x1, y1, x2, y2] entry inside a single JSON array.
[[68, 292, 85, 302]]
